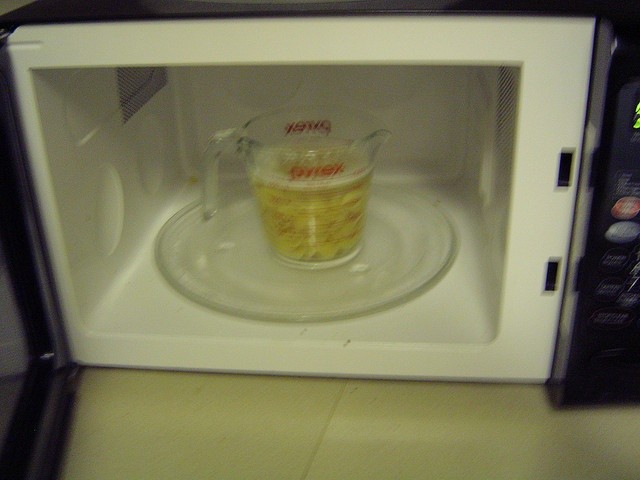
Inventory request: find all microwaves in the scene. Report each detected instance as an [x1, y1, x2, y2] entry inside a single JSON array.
[[0, 0, 639, 480]]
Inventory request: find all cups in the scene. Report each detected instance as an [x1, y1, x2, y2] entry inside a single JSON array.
[[200, 107, 395, 271]]
